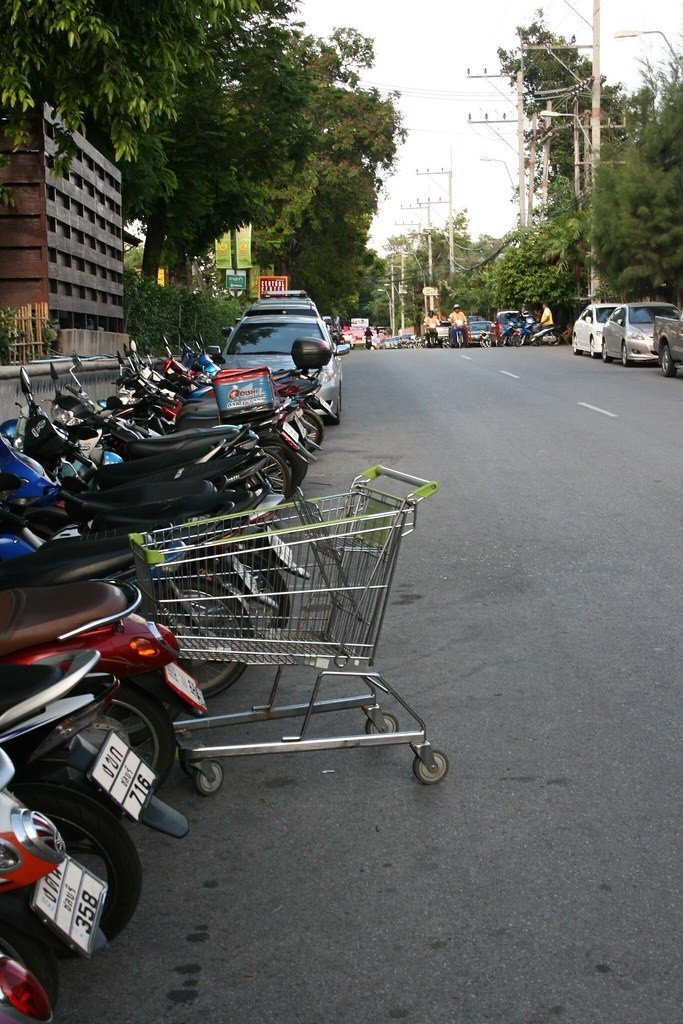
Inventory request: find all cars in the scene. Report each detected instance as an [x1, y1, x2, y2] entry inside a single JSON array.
[[207, 289, 355, 424], [380, 310, 520, 349], [572, 304, 627, 358], [602, 302, 682, 367]]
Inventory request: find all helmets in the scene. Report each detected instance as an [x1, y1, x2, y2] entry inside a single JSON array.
[[454, 304, 460, 311], [523, 311, 530, 317], [428, 310, 434, 316]]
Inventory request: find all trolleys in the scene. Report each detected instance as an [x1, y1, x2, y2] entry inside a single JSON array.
[[126, 464, 450, 795]]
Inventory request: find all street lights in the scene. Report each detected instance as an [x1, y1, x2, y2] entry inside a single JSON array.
[[384, 284, 404, 334], [378, 289, 395, 336], [540, 110, 601, 304], [404, 252, 434, 314], [423, 226, 455, 283], [480, 157, 525, 244]]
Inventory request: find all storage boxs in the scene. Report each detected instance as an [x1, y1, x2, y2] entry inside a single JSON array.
[[211, 363, 276, 421]]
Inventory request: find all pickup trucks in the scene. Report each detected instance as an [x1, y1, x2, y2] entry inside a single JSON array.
[[653, 308, 683, 376]]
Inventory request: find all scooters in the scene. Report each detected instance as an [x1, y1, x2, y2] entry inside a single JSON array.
[[500, 317, 561, 346], [0, 748, 113, 1023], [0, 579, 209, 795], [447, 316, 468, 348], [422, 320, 442, 347], [1, 649, 189, 943], [0, 327, 332, 698]]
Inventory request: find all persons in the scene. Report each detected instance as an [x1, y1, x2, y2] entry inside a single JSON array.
[[424, 310, 441, 347], [343, 324, 349, 332], [374, 325, 379, 334], [448, 304, 468, 348], [364, 327, 373, 344], [539, 303, 559, 345]]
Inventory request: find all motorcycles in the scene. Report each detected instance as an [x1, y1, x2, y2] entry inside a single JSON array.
[[364, 330, 374, 350]]
